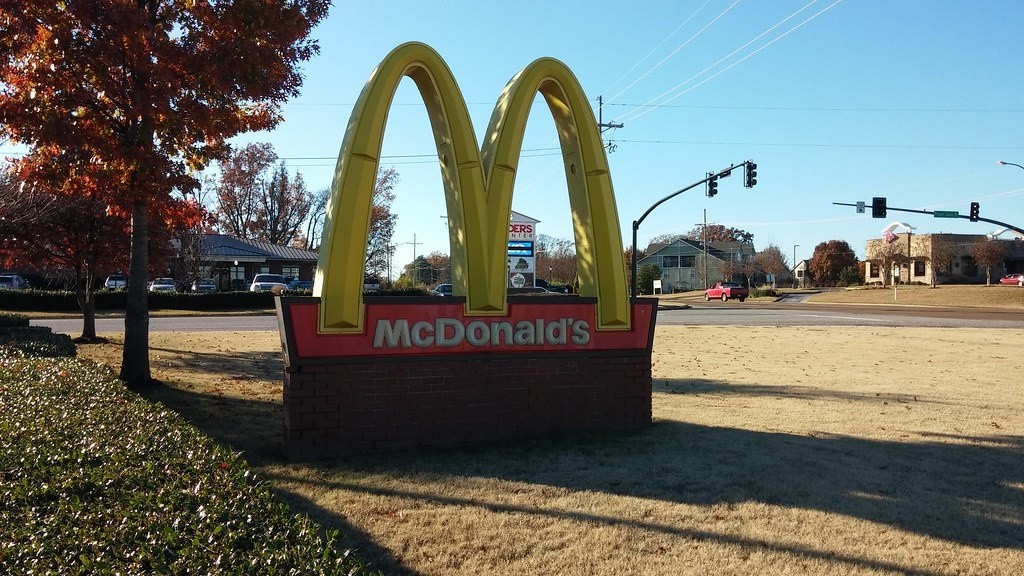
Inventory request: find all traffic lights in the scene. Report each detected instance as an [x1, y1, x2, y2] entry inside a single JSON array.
[[745, 160, 757, 187], [871, 196, 887, 218], [705, 171, 718, 197], [970, 202, 979, 222]]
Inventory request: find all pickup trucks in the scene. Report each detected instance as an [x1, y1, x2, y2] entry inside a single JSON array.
[[703, 281, 749, 302]]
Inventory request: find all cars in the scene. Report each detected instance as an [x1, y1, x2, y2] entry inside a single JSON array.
[[147, 277, 178, 293], [245, 274, 290, 293], [432, 283, 452, 297], [190, 278, 217, 293], [287, 280, 312, 290], [999, 274, 1024, 286], [536, 278, 568, 294], [105, 274, 127, 291], [363, 278, 380, 294]]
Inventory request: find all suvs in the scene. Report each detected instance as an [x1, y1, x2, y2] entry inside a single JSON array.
[[0, 272, 33, 291]]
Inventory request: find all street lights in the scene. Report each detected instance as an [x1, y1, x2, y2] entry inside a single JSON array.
[[792, 244, 800, 288], [549, 265, 552, 284]]
